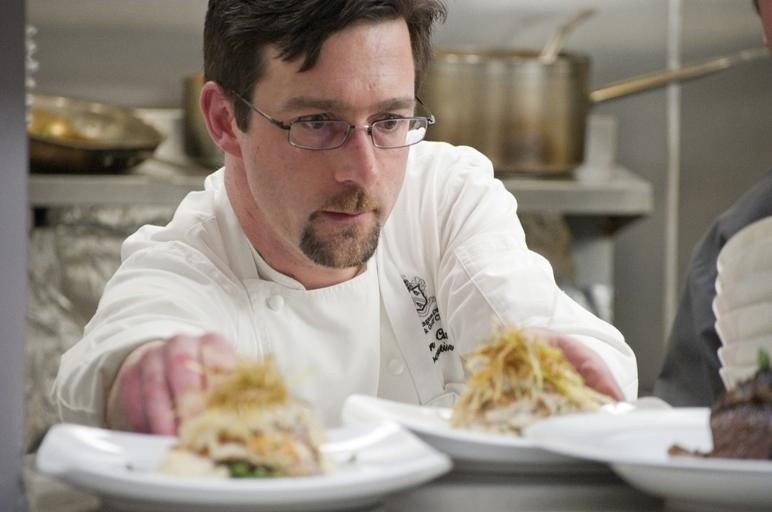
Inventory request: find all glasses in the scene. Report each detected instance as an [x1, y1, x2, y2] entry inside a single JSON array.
[[230, 88, 436, 151]]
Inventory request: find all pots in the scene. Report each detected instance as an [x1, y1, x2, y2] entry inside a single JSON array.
[[425, 50, 772, 178]]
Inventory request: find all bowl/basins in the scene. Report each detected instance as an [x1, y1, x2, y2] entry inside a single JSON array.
[[27, 97, 162, 173]]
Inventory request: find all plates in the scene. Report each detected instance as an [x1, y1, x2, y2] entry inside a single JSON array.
[[711, 216, 771, 390], [349, 395, 676, 476], [34, 412, 455, 510], [524, 405, 771, 508]]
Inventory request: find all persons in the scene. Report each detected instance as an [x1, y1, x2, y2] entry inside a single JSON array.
[[652, 0, 772, 407], [49, 0, 638, 436]]
